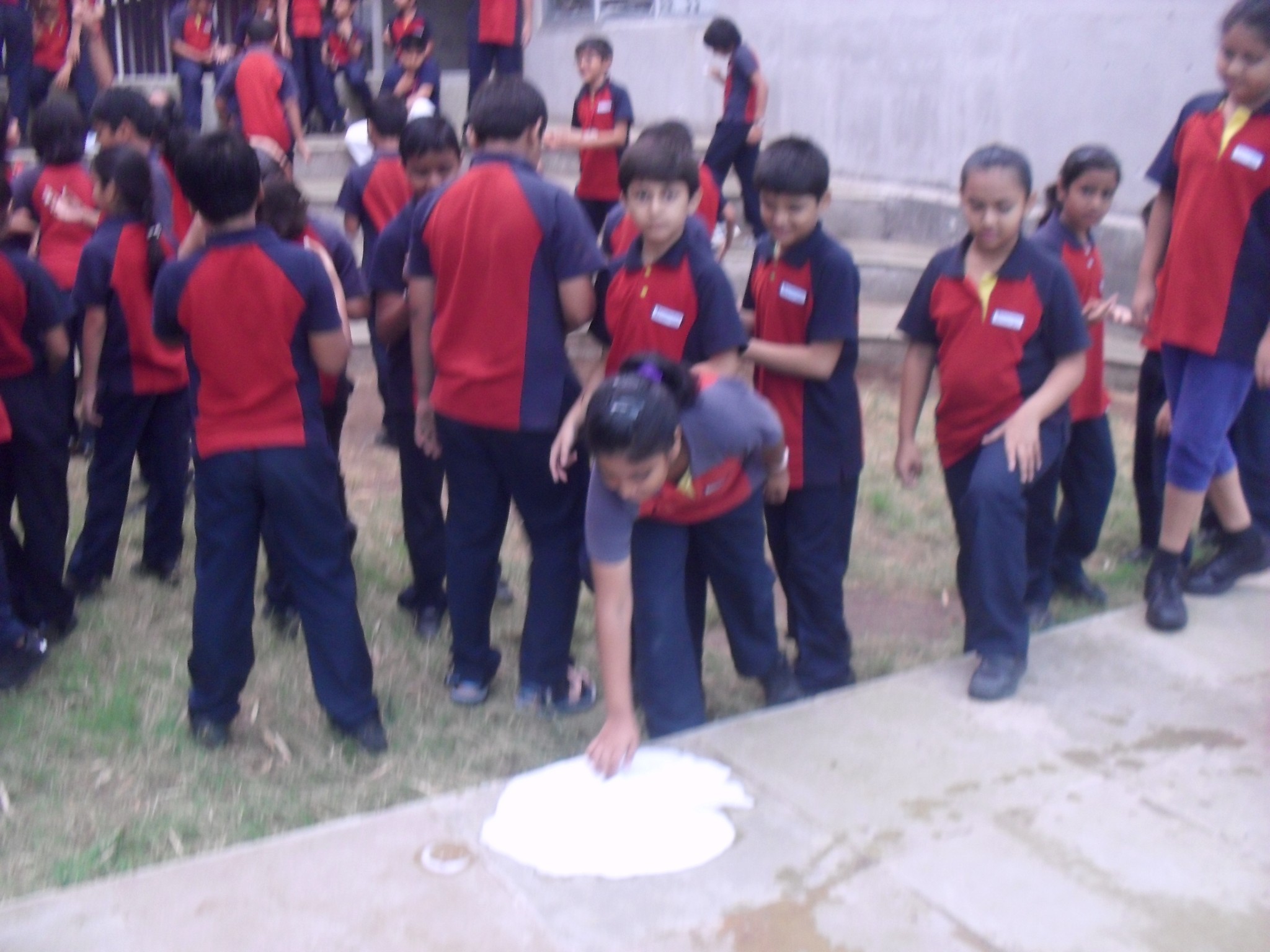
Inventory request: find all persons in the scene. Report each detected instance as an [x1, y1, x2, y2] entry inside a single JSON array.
[[0, 408, 51, 698], [548, 136, 750, 705], [365, 115, 523, 621], [153, 131, 387, 759], [579, 348, 803, 781], [0, 2, 771, 474], [0, 170, 81, 639], [1023, 145, 1124, 630], [890, 142, 1092, 705], [732, 131, 868, 699], [1105, 0, 1270, 633], [406, 80, 619, 714], [59, 141, 195, 594], [207, 143, 373, 633]]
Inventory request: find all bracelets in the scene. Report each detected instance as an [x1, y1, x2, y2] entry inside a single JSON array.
[[768, 445, 790, 472]]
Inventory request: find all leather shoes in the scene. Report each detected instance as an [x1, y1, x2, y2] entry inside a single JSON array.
[[1185, 528, 1270, 594], [967, 653, 1028, 701], [1144, 564, 1188, 631], [1057, 575, 1107, 607]]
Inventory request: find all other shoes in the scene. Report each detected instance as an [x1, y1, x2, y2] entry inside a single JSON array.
[[61, 421, 854, 739]]
